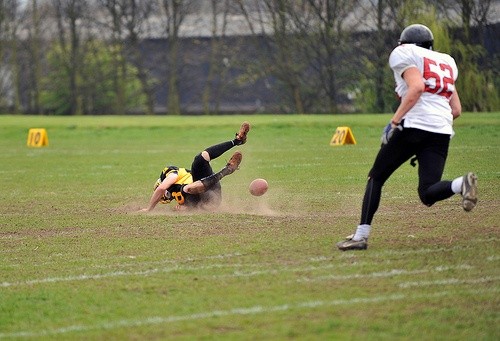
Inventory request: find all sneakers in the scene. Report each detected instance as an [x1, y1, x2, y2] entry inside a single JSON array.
[[337, 234, 367, 250], [236, 121, 249, 144], [462, 173, 479, 212], [227, 151, 242, 174]]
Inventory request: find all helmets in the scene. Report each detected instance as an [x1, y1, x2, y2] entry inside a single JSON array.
[[154, 179, 172, 204], [399, 23, 433, 45]]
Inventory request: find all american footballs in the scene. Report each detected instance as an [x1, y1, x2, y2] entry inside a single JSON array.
[[249, 179, 268, 196]]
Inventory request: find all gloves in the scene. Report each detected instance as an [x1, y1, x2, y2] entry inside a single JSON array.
[[382, 123, 397, 146]]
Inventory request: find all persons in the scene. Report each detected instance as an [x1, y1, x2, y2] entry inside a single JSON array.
[[137, 122, 250, 214], [337, 25, 478, 251]]
